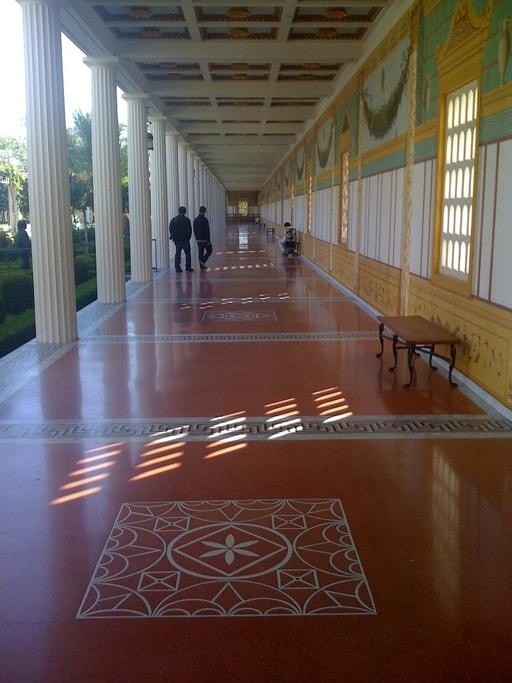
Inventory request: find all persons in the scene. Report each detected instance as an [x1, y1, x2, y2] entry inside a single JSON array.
[[278, 222, 296, 256], [174, 271, 192, 327], [169, 206, 194, 272], [193, 205, 212, 269], [195, 268, 213, 326]]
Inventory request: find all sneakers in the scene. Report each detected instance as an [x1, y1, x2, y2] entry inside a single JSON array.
[[176, 268, 183, 272], [186, 267, 195, 272], [199, 263, 208, 271]]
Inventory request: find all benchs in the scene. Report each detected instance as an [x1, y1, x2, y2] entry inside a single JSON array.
[[250, 215, 299, 256], [375, 313, 463, 388]]
